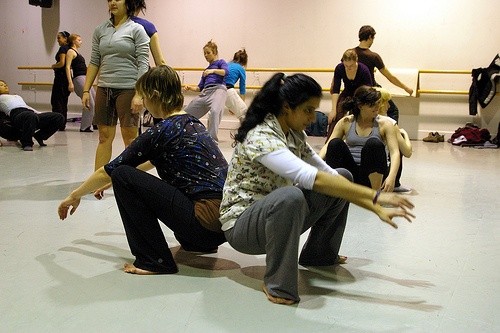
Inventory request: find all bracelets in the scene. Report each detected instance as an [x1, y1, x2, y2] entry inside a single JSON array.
[[372, 188, 381, 205], [82, 90, 90, 95]]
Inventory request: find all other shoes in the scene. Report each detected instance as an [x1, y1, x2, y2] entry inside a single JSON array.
[[435, 132, 444, 142], [423, 132, 439, 142]]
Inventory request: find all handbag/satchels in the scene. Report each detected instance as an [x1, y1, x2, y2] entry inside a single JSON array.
[[447, 122, 491, 145], [306, 111, 328, 136]]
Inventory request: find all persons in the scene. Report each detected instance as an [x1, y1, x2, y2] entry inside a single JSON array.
[[58, 66, 230, 275], [0, 0, 417, 195], [216, 71, 418, 305]]
